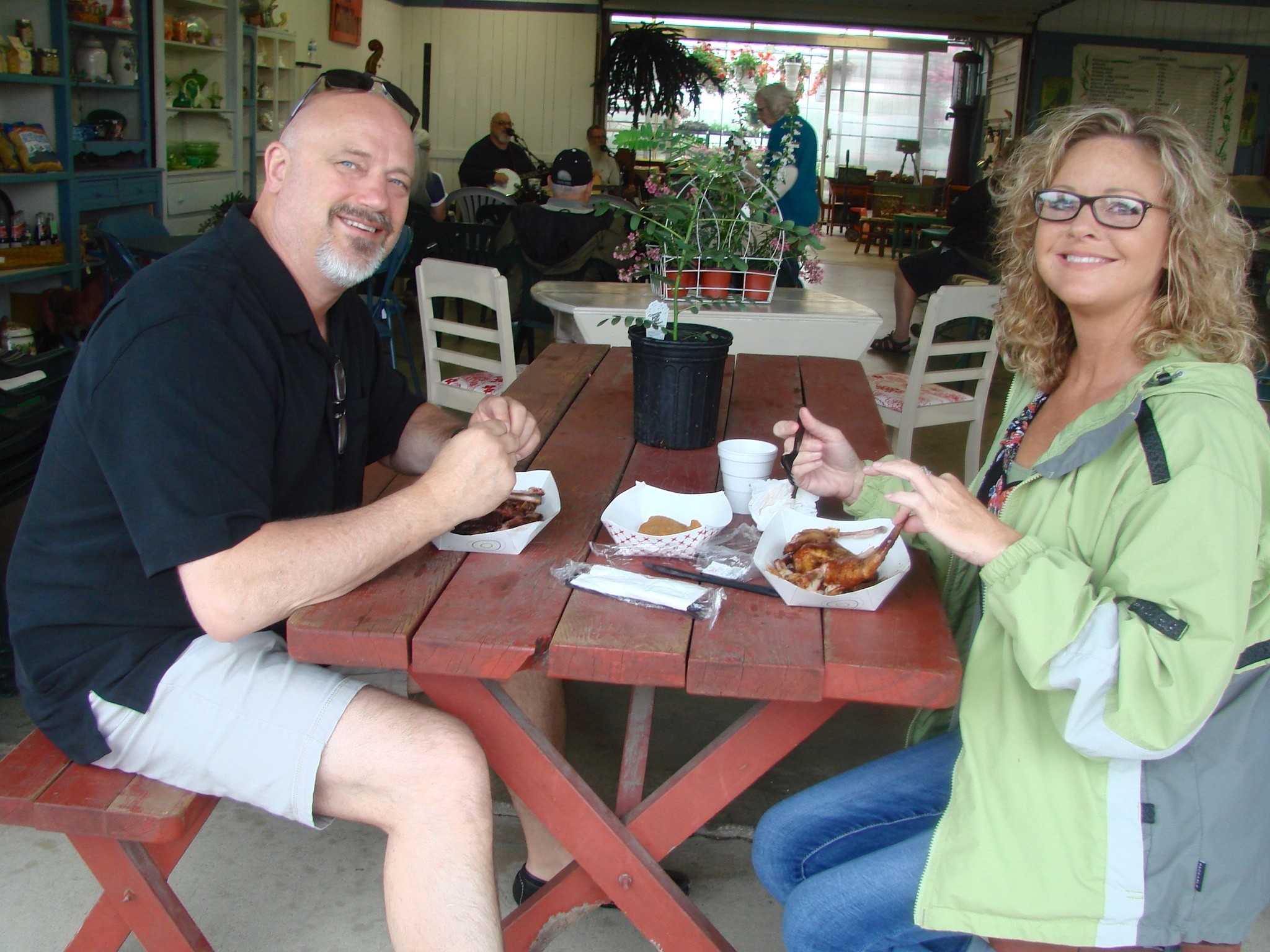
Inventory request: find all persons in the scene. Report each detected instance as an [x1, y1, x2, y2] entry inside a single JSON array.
[[753, 83, 820, 288], [458, 111, 538, 265], [6, 74, 690, 951], [583, 125, 636, 209], [869, 138, 1025, 354], [513, 148, 615, 328], [748, 96, 1270, 952], [407, 127, 449, 249]]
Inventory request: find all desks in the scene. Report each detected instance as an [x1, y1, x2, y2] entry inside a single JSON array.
[[287, 344, 961, 952], [890, 213, 946, 259], [531, 280, 882, 360], [828, 183, 870, 236]]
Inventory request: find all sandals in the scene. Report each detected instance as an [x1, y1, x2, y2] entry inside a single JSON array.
[[870, 330, 911, 353], [910, 322, 938, 340]]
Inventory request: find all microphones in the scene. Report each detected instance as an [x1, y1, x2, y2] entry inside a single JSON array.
[[601, 145, 608, 151], [507, 128, 515, 136]]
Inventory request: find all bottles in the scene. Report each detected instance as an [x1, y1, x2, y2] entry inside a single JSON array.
[[0, 210, 59, 248]]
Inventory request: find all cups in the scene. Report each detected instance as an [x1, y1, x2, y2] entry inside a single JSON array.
[[528, 178, 541, 191], [718, 439, 779, 514]]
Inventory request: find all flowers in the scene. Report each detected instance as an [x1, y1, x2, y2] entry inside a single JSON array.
[[679, 39, 854, 103], [613, 178, 829, 286]]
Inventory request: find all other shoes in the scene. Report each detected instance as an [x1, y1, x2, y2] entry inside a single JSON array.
[[512, 855, 691, 909]]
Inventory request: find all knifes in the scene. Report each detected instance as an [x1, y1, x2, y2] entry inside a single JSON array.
[[643, 562, 780, 597]]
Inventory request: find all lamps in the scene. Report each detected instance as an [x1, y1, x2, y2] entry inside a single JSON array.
[[950, 50, 982, 118]]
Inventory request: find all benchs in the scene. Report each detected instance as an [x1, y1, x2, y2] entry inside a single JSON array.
[[0, 460, 425, 952]]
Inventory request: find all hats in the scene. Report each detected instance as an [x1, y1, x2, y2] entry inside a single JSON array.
[[725, 134, 746, 145], [552, 148, 595, 187]]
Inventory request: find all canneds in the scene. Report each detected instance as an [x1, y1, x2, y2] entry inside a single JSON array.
[[16, 18, 60, 77]]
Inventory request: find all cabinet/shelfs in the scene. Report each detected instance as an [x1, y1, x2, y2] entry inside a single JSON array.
[[0, 0, 296, 505]]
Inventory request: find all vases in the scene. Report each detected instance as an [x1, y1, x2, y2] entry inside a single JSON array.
[[661, 268, 775, 302], [783, 62, 802, 91], [735, 64, 750, 76]]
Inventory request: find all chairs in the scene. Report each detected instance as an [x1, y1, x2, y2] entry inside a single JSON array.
[[867, 263, 1005, 488], [352, 186, 617, 414], [814, 176, 971, 260]]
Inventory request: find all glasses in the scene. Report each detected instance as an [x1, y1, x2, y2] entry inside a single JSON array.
[[278, 69, 421, 143], [325, 354, 350, 459], [1033, 189, 1171, 229], [757, 107, 764, 111], [493, 120, 514, 127]]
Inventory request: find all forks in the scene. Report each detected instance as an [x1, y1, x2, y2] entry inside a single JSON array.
[[780, 404, 807, 487]]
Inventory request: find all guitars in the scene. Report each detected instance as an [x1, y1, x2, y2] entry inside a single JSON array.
[[548, 170, 639, 195], [486, 167, 552, 200]]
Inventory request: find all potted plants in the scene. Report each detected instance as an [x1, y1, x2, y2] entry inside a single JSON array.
[[589, 123, 825, 450]]
